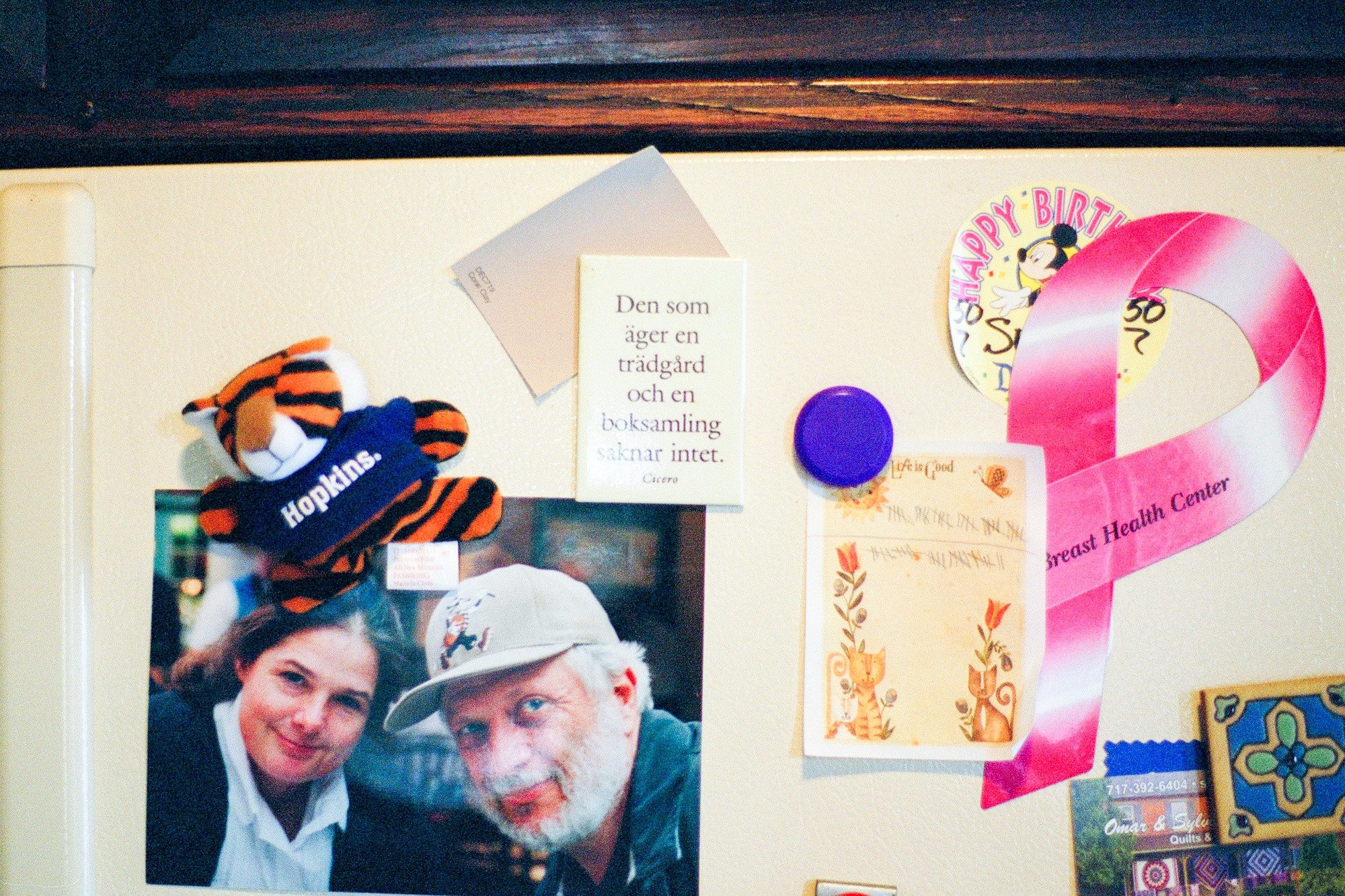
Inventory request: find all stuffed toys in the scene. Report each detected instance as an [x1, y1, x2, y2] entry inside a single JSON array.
[[176, 339, 502, 614]]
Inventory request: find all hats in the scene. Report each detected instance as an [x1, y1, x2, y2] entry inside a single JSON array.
[[383, 563, 623, 734]]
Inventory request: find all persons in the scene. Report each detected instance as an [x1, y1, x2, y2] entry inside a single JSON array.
[[146, 531, 443, 895], [381, 560, 700, 896]]
